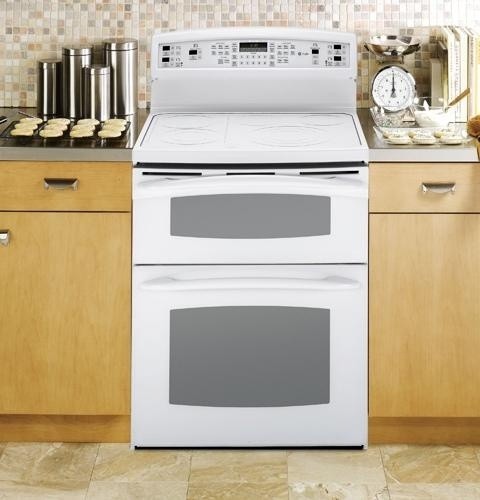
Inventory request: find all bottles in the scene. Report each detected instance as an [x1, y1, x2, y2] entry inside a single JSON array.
[[35, 36, 141, 122]]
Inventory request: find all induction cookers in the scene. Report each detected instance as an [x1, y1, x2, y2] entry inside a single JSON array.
[[131, 110, 369, 164]]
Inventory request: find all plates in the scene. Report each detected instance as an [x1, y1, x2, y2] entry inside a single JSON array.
[[372, 126, 475, 145]]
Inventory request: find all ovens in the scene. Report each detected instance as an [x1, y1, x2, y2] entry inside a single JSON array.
[[131, 266, 368, 447], [131, 168, 368, 265]]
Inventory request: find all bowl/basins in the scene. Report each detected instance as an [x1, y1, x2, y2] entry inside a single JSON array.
[[409, 94, 458, 127], [368, 104, 409, 127]]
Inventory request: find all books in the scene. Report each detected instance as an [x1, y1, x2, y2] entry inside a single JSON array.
[[438, 26, 479, 123]]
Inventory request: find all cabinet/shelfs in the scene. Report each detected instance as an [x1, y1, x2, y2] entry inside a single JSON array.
[[1, 159, 132, 443], [368, 161, 480, 447]]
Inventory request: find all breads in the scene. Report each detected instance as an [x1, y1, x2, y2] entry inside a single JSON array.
[[382, 130, 461, 144], [10, 117, 128, 138]]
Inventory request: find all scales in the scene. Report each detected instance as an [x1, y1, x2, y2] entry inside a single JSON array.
[[364, 35, 422, 122]]
